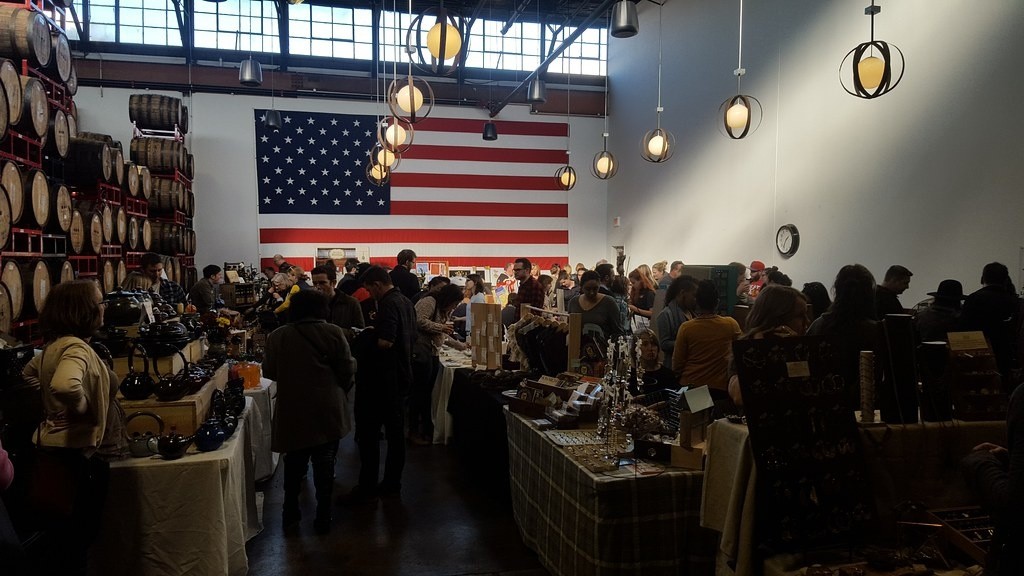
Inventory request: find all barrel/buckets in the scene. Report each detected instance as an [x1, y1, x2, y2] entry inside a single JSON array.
[[0, 258, 103, 334], [112, 140, 151, 199], [0, 161, 155, 249], [0, 5, 78, 96], [99, 259, 128, 302], [148, 177, 195, 217], [149, 223, 196, 255], [129, 94, 188, 136], [0, 58, 112, 180]]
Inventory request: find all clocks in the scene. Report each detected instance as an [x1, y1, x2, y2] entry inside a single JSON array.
[[776, 225, 800, 258]]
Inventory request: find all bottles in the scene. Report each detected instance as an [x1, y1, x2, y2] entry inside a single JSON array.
[[177, 301, 184, 314], [226, 266, 242, 283], [236, 288, 254, 305], [130, 136, 195, 179], [231, 364, 238, 380], [243, 307, 261, 338], [624, 434, 634, 452]]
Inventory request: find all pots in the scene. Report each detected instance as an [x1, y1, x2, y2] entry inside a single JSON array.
[[106, 286, 176, 326]]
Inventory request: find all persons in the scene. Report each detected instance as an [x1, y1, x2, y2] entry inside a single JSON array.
[[121, 271, 152, 291], [17, 280, 128, 576], [189, 264, 222, 315], [247, 249, 464, 536], [499, 258, 1024, 576], [141, 252, 186, 305], [465, 274, 486, 333]]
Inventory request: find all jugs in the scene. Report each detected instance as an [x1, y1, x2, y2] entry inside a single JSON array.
[[89, 314, 246, 459]]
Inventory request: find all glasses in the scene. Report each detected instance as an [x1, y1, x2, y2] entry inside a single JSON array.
[[583, 286, 599, 292], [512, 268, 525, 272], [782, 310, 808, 324]]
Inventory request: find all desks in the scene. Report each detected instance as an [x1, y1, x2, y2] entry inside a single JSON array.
[[113, 397, 253, 576], [504, 404, 704, 576]]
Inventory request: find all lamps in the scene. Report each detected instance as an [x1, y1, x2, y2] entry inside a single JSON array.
[[528, 81, 546, 103], [406, 8, 471, 74], [239, 59, 264, 87], [838, 6, 904, 97], [387, 75, 435, 124], [266, 110, 282, 131], [377, 117, 414, 153], [639, 107, 676, 164], [555, 151, 577, 191], [366, 160, 390, 187], [370, 142, 401, 172], [611, 1, 638, 38], [483, 124, 497, 140], [717, 68, 763, 140], [589, 133, 619, 180]]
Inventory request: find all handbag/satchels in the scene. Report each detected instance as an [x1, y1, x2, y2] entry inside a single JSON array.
[[27, 447, 80, 509], [330, 354, 354, 393]]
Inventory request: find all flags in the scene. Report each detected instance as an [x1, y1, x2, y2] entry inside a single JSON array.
[[253, 108, 570, 272]]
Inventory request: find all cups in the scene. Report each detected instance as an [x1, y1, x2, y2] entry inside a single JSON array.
[[238, 362, 260, 387], [445, 321, 454, 334]]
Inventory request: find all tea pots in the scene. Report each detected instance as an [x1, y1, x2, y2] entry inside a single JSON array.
[[158, 255, 197, 292]]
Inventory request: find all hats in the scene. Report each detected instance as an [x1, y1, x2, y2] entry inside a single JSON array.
[[928, 280, 971, 301], [750, 261, 765, 271]]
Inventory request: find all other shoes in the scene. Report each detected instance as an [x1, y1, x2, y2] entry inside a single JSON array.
[[404, 430, 419, 446], [283, 517, 300, 537], [314, 511, 337, 536], [301, 470, 307, 480], [351, 484, 376, 499], [414, 434, 433, 448], [372, 474, 401, 497]]
